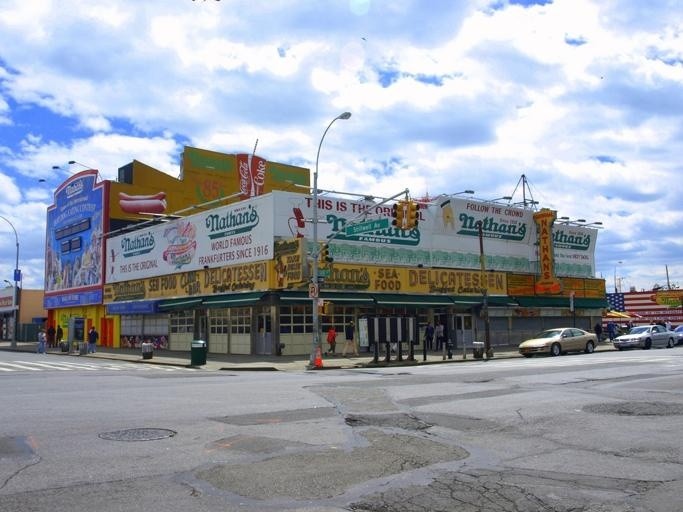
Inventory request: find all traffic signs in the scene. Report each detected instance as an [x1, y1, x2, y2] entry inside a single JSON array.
[[345, 218, 388, 238]]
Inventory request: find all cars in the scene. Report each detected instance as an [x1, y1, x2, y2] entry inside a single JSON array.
[[675, 324, 683, 344], [613, 325, 679, 349], [518, 327, 599, 356]]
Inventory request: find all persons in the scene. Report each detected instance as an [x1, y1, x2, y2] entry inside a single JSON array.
[[47, 325, 56, 348], [424, 322, 434, 350], [341, 320, 359, 357], [434, 320, 444, 352], [56, 324, 63, 347], [36, 327, 48, 357], [594, 319, 672, 343], [87, 326, 99, 354], [325, 327, 336, 356]]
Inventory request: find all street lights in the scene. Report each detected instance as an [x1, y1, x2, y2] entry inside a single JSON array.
[[308, 112, 352, 370], [614, 261, 622, 293]]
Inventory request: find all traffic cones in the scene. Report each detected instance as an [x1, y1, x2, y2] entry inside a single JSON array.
[[314, 347, 324, 368]]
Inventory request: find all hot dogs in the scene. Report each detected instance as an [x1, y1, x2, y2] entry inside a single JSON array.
[[119, 188, 167, 214]]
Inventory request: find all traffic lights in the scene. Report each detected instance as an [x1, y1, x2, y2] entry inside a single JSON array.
[[391, 198, 403, 229], [326, 303, 333, 313], [321, 240, 329, 262], [406, 199, 419, 230]]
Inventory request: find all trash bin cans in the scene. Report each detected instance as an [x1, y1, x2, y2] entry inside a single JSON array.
[[142, 342, 153, 359], [191, 340, 208, 365], [78, 342, 88, 355], [61, 342, 69, 352], [472, 341, 485, 358]]
